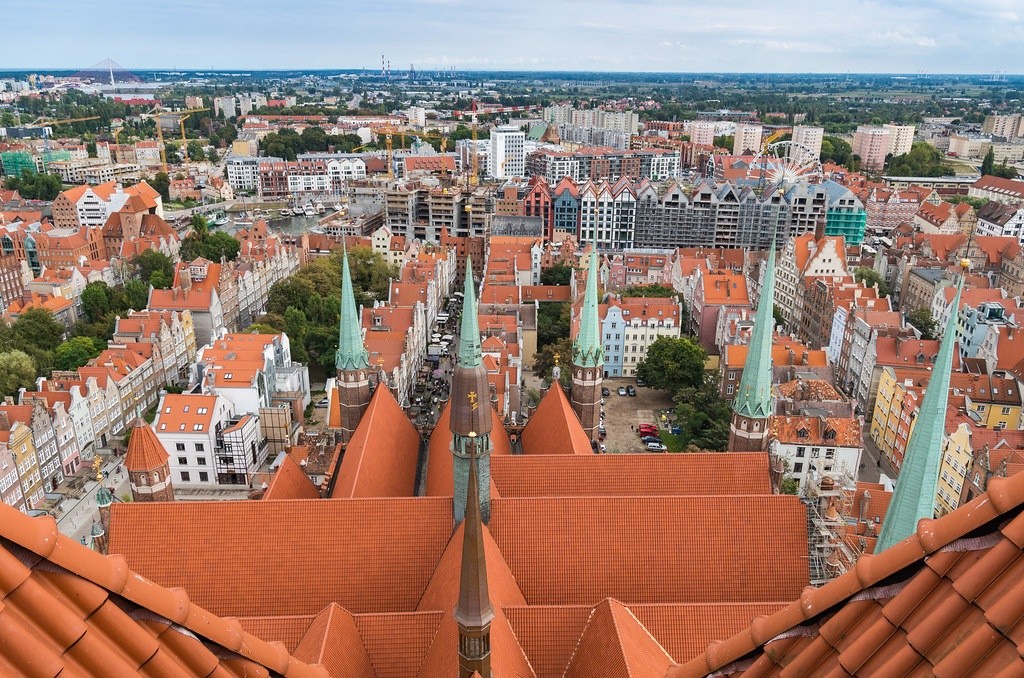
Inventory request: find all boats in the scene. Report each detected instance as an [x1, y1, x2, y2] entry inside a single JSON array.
[[208, 204, 328, 228]]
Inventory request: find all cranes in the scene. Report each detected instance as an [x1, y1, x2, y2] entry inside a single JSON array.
[[757, 128, 784, 193], [372, 120, 448, 180], [34, 115, 101, 157], [453, 101, 539, 184], [154, 106, 212, 177]]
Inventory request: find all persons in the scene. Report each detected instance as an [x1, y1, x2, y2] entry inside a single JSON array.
[[636, 428, 639, 436], [631, 424, 633, 432], [661, 409, 665, 416]]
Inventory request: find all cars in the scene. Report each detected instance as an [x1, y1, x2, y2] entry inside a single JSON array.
[[599, 411, 607, 452], [639, 407, 682, 452], [617, 386, 626, 396], [636, 376, 646, 387], [626, 385, 637, 397], [314, 396, 327, 408], [602, 387, 610, 397]]
